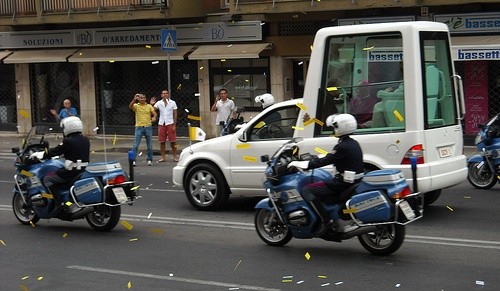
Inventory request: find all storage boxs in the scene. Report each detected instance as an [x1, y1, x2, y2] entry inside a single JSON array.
[[360, 167, 404, 189], [73, 177, 102, 205], [82, 159, 123, 177], [350, 189, 391, 223]]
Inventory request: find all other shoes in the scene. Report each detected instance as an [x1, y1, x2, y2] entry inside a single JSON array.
[[172, 157, 179, 161], [147, 160, 153, 166], [159, 158, 166, 162], [133, 161, 136, 166]]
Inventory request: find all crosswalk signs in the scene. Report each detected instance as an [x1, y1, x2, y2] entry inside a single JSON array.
[[162, 29, 177, 52]]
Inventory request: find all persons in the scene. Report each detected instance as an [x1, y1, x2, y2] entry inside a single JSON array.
[[286, 113, 364, 236], [372, 61, 404, 128], [129, 92, 158, 166], [211, 89, 237, 138], [50, 99, 78, 122], [321, 78, 341, 131], [28, 115, 90, 218], [255, 93, 281, 125], [153, 88, 181, 162], [347, 78, 378, 129]]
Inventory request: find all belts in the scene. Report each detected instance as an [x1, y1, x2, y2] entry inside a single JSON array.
[[73, 162, 89, 167], [340, 172, 365, 180]]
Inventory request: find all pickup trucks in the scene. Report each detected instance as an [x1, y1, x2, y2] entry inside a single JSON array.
[[169, 17, 469, 213]]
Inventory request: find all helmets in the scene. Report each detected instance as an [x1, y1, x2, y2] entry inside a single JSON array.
[[334, 113, 357, 137], [259, 93, 274, 109], [61, 115, 83, 134]]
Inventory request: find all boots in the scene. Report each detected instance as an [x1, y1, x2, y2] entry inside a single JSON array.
[[47, 183, 66, 214], [306, 197, 336, 237]]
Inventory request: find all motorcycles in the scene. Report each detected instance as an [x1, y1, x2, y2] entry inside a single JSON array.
[[254, 136, 425, 257], [11, 124, 140, 233], [465, 112, 500, 192]]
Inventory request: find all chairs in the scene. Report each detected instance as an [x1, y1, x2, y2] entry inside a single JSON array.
[[375, 66, 446, 125]]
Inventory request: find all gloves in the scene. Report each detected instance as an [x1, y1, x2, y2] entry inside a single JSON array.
[[287, 160, 311, 170], [29, 150, 46, 160], [317, 153, 326, 158]]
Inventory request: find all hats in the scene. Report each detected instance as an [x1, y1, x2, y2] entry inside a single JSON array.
[[328, 78, 341, 88], [356, 80, 369, 96]]
